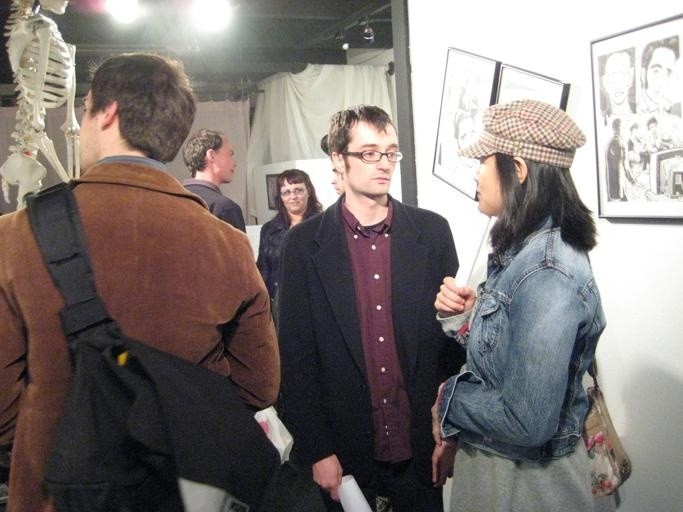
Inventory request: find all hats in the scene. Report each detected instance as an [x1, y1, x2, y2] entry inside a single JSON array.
[[461, 98, 586, 167]]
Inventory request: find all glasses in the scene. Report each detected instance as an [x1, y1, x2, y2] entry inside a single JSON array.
[[340, 148, 404, 165]]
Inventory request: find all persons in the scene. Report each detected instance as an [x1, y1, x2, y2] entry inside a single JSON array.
[[321, 135, 346, 197], [273, 105, 471, 510], [254, 170, 329, 300], [601, 35, 682, 203], [430, 100, 623, 510], [172, 131, 249, 237], [0, 52, 282, 512]]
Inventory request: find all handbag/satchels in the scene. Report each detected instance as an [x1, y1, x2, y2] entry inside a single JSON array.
[[29, 198, 291, 511], [451, 380, 631, 508]]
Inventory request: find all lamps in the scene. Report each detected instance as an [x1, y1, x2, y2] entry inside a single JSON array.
[[360, 15, 376, 40], [334, 32, 350, 51]]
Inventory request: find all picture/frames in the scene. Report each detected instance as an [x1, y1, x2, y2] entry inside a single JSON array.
[[265, 173, 281, 210], [590, 15, 683, 220], [495, 63, 570, 111], [432, 46, 502, 202]]
